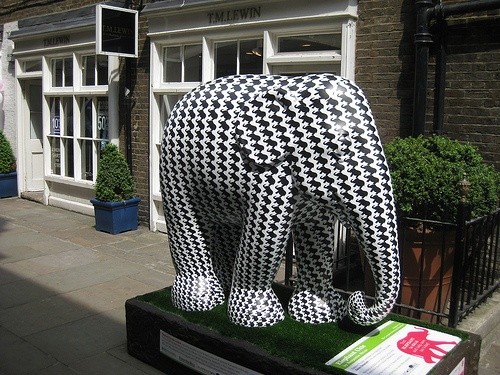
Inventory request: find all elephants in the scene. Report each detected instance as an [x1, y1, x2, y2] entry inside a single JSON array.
[[159, 73, 400, 327], [397, 327, 457, 363]]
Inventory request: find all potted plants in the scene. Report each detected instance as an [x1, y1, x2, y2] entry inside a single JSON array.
[[0, 132, 18, 199], [89, 143, 143, 236], [380, 134, 499, 321]]
